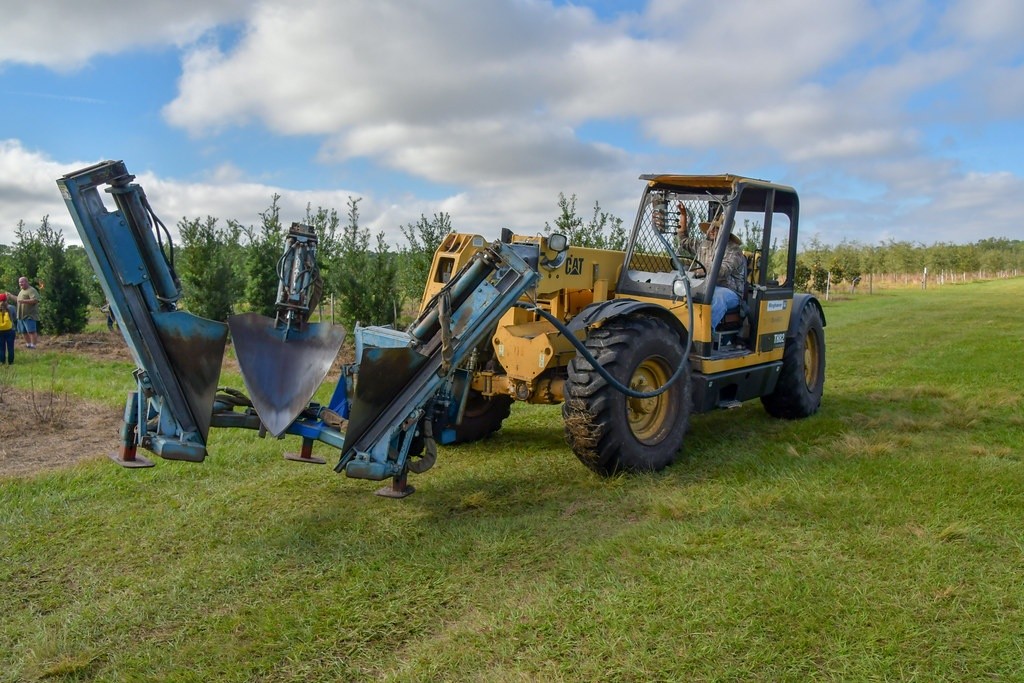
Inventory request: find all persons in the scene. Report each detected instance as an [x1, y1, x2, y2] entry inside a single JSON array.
[[6, 276, 42, 349], [676, 199, 746, 330], [0, 293, 17, 366], [107, 305, 122, 335]]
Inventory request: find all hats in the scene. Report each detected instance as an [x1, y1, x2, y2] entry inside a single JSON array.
[[698, 213, 743, 245], [0, 294, 7, 301]]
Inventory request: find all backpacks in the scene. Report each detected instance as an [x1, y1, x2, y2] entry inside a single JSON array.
[[0, 311, 12, 331]]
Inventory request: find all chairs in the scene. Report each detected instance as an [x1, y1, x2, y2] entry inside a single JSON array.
[[716, 282, 748, 331]]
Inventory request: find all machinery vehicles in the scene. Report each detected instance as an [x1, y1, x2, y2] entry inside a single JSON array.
[[56, 159, 827, 498]]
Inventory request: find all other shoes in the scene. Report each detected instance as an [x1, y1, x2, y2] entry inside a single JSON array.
[[30, 346, 36, 349], [26, 345, 30, 348]]
